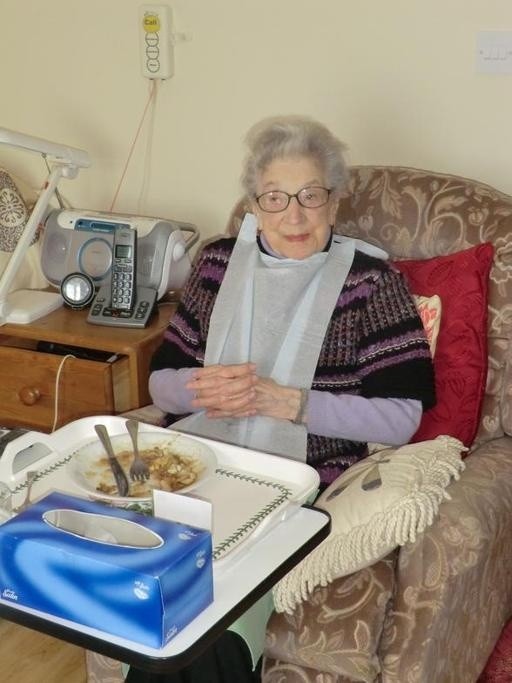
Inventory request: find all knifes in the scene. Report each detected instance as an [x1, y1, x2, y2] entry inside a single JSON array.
[[92, 423, 130, 498]]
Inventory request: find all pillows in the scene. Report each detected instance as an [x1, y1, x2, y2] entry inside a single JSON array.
[[389, 242, 494, 451], [272, 436, 468, 613]]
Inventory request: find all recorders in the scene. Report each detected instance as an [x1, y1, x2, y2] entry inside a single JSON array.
[[38, 207, 200, 301]]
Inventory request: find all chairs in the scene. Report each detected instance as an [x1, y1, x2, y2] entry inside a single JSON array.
[[88, 165, 512, 683], [1, 130, 92, 326]]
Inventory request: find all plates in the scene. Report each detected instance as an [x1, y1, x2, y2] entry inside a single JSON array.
[[64, 428, 220, 505]]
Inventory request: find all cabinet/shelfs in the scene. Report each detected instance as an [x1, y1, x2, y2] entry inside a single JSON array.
[[2, 283, 182, 438]]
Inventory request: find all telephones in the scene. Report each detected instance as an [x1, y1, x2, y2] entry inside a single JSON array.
[[86, 224, 158, 327]]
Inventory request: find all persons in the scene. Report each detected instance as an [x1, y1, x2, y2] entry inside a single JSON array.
[[123, 112, 437, 682]]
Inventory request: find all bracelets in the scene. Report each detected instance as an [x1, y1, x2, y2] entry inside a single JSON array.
[[295, 388, 308, 425]]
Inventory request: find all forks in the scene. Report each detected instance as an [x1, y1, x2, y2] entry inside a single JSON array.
[[123, 415, 156, 485]]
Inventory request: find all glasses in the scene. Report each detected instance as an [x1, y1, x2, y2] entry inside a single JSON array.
[[254, 187, 332, 213]]
